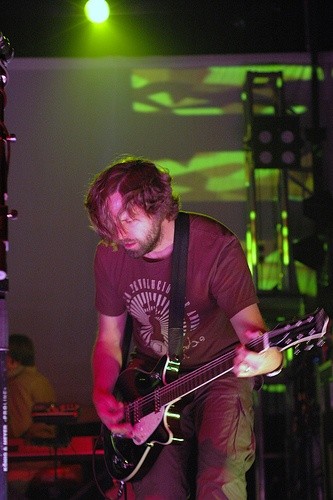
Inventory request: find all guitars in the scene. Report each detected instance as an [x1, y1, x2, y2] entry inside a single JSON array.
[[101, 308, 329, 484]]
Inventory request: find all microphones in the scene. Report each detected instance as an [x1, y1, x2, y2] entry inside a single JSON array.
[[110, 437, 130, 466], [0, 32, 13, 64]]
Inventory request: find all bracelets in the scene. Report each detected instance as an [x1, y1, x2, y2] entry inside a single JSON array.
[[264, 355, 286, 378]]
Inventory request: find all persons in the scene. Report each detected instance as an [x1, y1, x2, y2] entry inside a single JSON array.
[[83, 159, 284, 500], [3, 339, 66, 483]]
